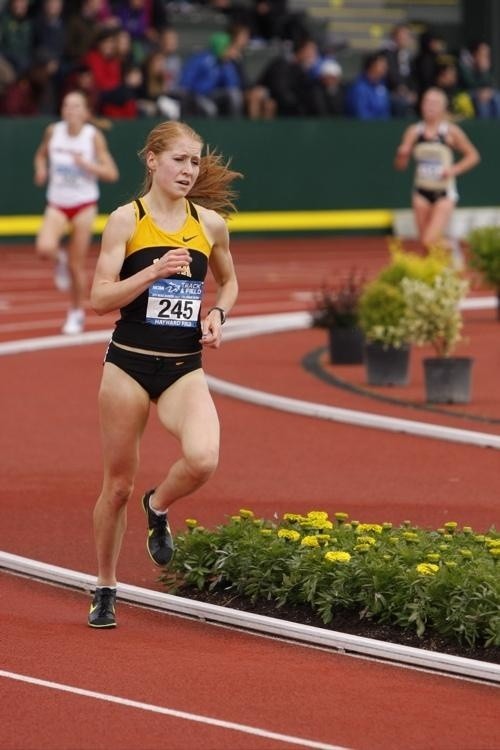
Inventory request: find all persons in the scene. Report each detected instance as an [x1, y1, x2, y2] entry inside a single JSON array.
[[86, 118, 246, 629], [33, 89, 120, 336], [0, 0, 498, 119], [393, 84, 481, 260]]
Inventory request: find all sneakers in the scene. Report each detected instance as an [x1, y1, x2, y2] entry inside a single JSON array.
[[142, 489, 174, 568], [88, 586, 117, 628], [54, 249, 85, 335]]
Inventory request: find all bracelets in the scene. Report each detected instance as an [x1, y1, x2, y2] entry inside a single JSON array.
[[206, 307, 226, 326]]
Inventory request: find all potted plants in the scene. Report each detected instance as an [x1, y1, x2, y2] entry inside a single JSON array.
[[304, 218, 500, 403]]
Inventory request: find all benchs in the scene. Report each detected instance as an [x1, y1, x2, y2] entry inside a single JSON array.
[[165, 0, 460, 83]]
